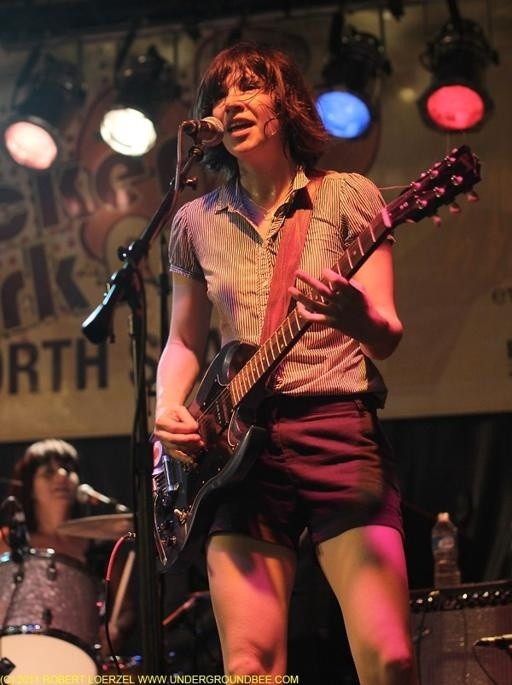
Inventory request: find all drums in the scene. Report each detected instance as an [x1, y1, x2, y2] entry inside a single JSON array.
[[162, 589, 337, 683], [0, 548, 103, 685]]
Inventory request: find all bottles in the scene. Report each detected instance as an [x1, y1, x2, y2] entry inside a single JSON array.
[[431, 511, 461, 587]]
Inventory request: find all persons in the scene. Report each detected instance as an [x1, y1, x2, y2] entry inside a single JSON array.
[[1, 437, 137, 666], [144, 40, 424, 684]]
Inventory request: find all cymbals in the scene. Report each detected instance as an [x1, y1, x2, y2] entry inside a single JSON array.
[[57, 513, 135, 541]]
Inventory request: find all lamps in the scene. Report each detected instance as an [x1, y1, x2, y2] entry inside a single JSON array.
[[101, 17, 182, 157], [315, 0, 396, 142], [3, 25, 85, 173], [416, 0, 500, 133]]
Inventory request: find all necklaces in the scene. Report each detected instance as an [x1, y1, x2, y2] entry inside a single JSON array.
[[241, 190, 291, 221]]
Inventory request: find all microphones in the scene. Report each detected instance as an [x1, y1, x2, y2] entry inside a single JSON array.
[[180, 117, 225, 146], [76, 483, 129, 513], [473, 634, 512, 650]]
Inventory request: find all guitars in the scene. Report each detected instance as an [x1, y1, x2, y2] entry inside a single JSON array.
[[151, 145, 480, 573]]
